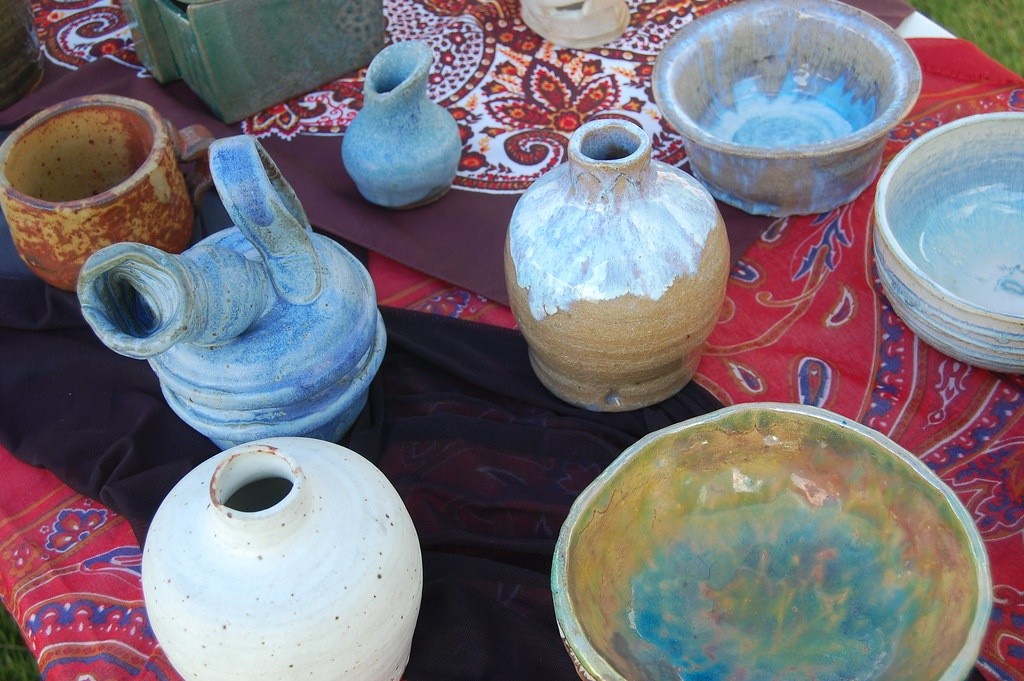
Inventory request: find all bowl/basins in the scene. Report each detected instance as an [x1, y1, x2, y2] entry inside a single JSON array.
[[650, 0, 924, 218], [547, 398, 1000, 681], [875, 113, 1023, 374]]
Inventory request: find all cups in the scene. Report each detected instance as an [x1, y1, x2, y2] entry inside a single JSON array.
[[0, 99, 216, 302]]
